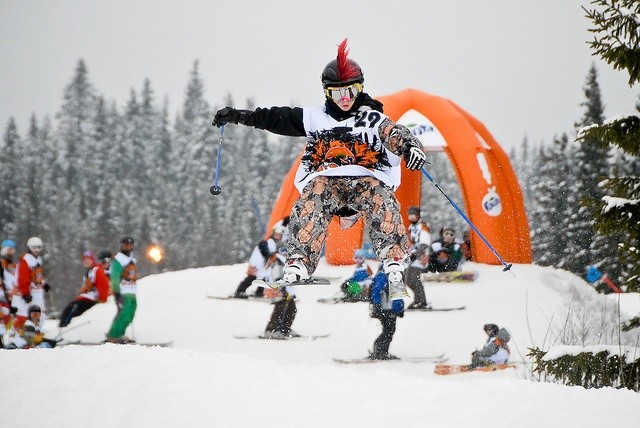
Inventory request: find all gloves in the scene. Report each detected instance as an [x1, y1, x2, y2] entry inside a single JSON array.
[[404, 145, 425, 171], [24, 294, 32, 303], [211, 106, 250, 128], [44, 283, 51, 292]]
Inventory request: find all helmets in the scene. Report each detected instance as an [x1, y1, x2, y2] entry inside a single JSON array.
[[407, 206, 420, 217], [28, 304, 41, 321], [97, 250, 112, 264], [484, 323, 499, 337], [321, 38, 364, 87], [82, 250, 95, 259], [27, 237, 43, 254], [119, 237, 135, 257]]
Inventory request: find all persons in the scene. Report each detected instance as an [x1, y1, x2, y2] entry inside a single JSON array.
[[342, 249, 374, 301], [368, 264, 399, 360], [263, 241, 298, 339], [58, 250, 108, 328], [430, 227, 457, 272], [0, 238, 57, 349], [405, 206, 430, 309], [98, 250, 112, 298], [232, 224, 283, 296], [101, 236, 136, 344], [460, 230, 472, 261], [471, 325, 509, 368], [211, 36, 426, 319]]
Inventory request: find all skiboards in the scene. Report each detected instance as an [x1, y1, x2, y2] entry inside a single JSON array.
[[251, 269, 420, 302], [206, 292, 300, 300], [333, 351, 447, 366], [62, 336, 173, 352], [434, 361, 518, 376], [387, 297, 470, 312], [231, 332, 333, 344]]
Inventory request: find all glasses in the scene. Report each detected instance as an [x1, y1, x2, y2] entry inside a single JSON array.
[[32, 247, 42, 252], [323, 83, 362, 104]]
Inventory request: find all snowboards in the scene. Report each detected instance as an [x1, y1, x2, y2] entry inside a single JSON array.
[[424, 269, 481, 286]]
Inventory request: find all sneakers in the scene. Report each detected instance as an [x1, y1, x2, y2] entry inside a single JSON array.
[[286, 261, 309, 279], [384, 259, 404, 273], [234, 290, 247, 299]]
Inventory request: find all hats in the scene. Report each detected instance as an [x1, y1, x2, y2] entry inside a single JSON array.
[[0, 239, 15, 247], [440, 225, 456, 234]]
